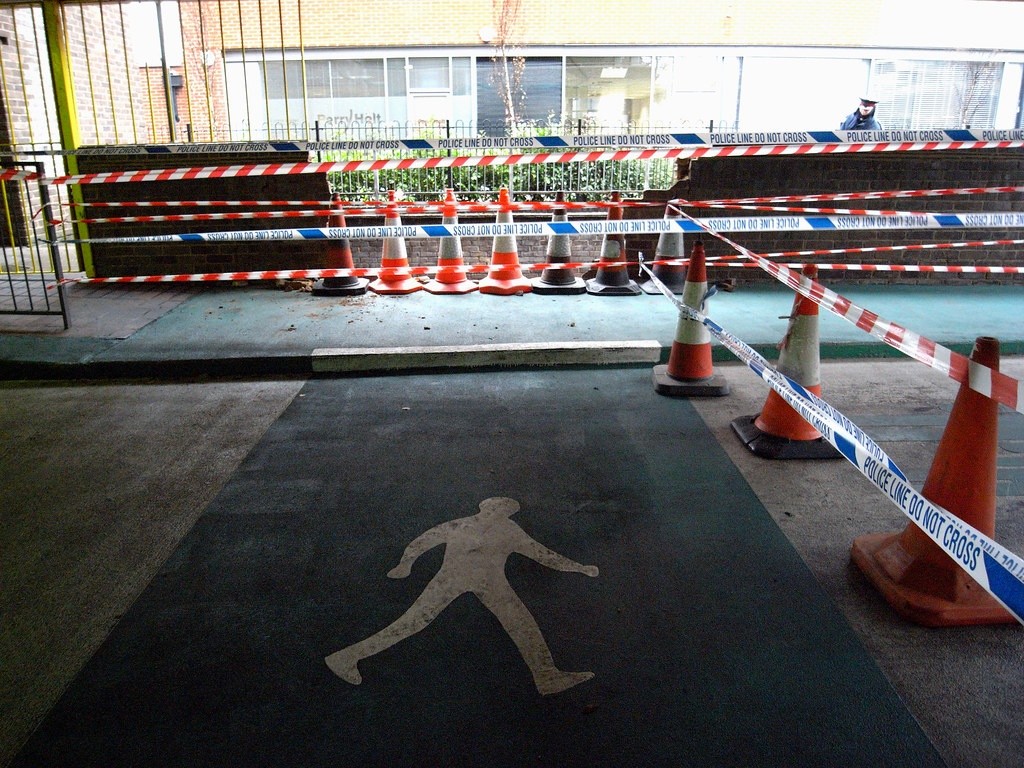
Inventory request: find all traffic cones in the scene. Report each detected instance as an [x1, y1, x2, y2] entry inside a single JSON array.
[[479, 185, 532, 296], [728, 259, 851, 459], [640, 200, 690, 296], [849, 334, 1024, 624], [368, 187, 422, 295], [586, 191, 643, 294], [310, 191, 370, 295], [651, 238, 732, 396], [531, 190, 588, 296], [423, 187, 469, 295]]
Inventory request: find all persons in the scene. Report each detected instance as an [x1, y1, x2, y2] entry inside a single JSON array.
[[839, 96, 883, 130]]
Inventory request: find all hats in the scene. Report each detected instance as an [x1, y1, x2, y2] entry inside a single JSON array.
[[859, 95, 879, 104]]
